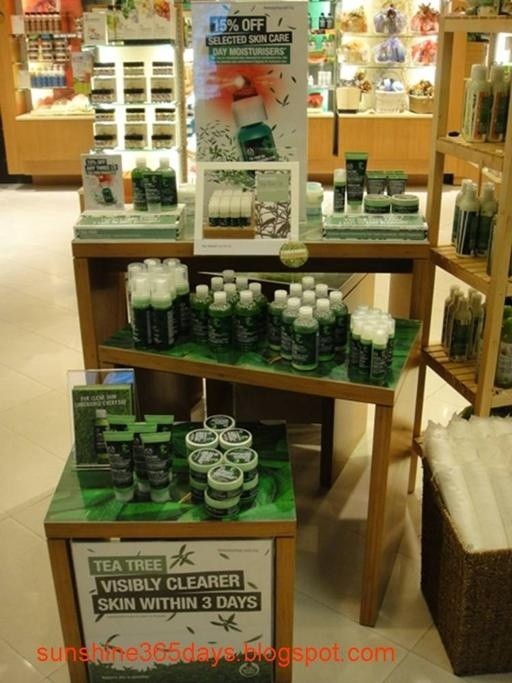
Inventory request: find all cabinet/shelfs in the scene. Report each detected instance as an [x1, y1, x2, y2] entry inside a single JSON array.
[[395, 0, 511, 445]]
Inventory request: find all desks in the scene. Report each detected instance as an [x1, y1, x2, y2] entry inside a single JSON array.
[[40, 187, 438, 683]]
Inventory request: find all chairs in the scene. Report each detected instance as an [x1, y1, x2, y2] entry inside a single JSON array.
[[0, 0, 487, 187]]
